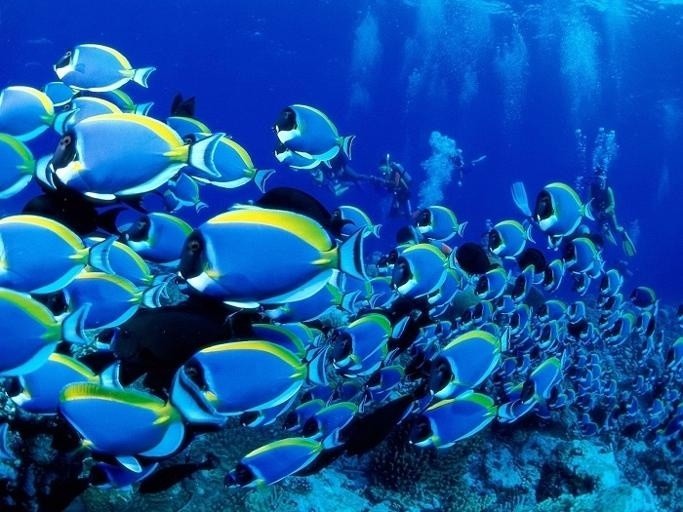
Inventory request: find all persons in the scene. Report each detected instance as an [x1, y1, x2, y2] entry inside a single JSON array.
[[586, 170, 637, 258], [314, 146, 491, 220]]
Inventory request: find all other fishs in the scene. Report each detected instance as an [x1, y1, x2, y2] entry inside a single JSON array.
[[1, 40, 683, 493]]
